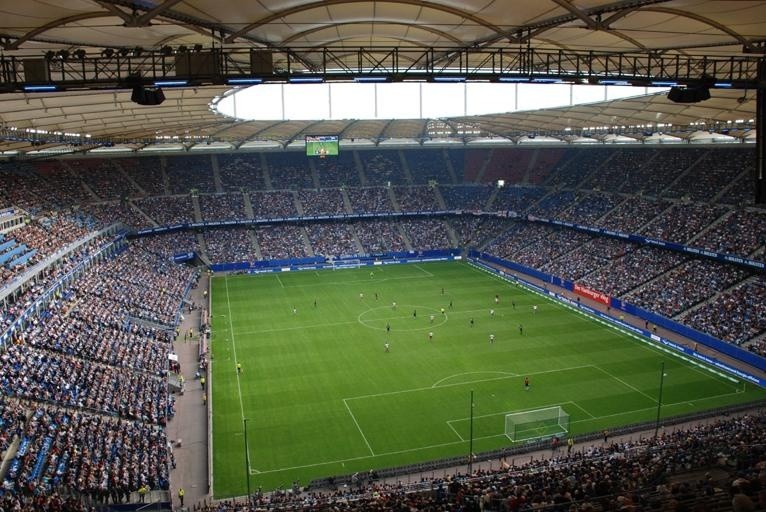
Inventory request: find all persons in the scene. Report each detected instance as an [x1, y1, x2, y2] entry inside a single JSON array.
[[187, 402, 765, 512], [384, 341, 389, 353], [235, 360, 242, 374], [392, 301, 397, 310], [469, 145, 766, 361], [412, 309, 417, 320], [441, 306, 446, 317], [313, 298, 317, 308], [440, 286, 446, 296], [359, 291, 364, 303], [207, 151, 469, 275], [448, 299, 455, 312], [429, 314, 435, 324], [428, 331, 434, 342], [523, 376, 530, 390], [369, 271, 376, 281], [292, 305, 297, 315], [374, 292, 379, 302], [386, 322, 391, 336], [1, 154, 208, 512]]
